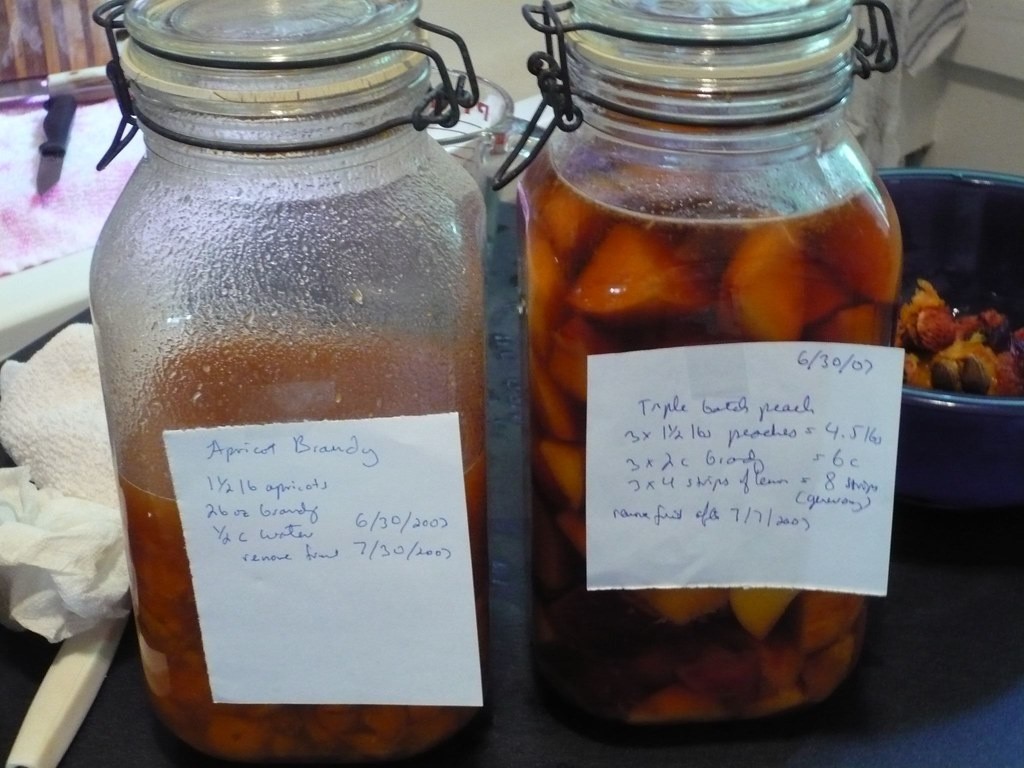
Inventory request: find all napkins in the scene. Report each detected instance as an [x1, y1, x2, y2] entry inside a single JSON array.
[[1, 322, 133, 643]]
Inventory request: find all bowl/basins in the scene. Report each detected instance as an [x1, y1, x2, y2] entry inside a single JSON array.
[[832, 166, 1024, 514]]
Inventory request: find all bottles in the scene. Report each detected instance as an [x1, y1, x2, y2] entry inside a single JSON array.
[[486, 5, 906, 739], [86, 1, 512, 763]]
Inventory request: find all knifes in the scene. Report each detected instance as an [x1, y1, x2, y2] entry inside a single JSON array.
[[34, 96, 78, 197]]
